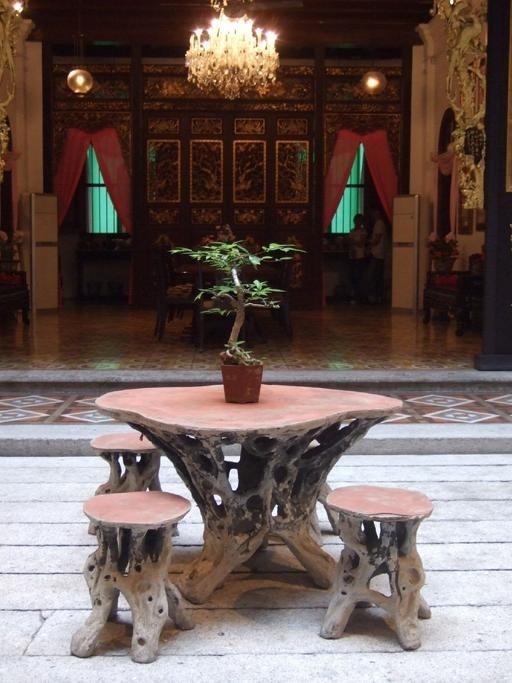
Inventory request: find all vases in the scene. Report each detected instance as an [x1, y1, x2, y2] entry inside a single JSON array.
[[434, 256, 456, 275]]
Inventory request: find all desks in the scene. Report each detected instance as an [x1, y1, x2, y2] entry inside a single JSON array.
[[94, 383, 402, 603]]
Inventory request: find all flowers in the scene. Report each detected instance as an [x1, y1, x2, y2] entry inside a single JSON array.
[[424, 232, 461, 257]]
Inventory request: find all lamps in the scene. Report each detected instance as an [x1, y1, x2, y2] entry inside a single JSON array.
[[66, 34, 93, 97], [358, 43, 387, 96], [184, 0, 281, 102], [10, 0, 25, 16]]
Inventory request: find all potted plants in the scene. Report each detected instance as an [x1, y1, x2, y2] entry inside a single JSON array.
[[168, 239, 306, 404]]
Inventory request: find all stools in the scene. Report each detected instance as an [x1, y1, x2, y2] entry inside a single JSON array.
[[319, 486, 432, 652], [88, 432, 180, 537], [71, 491, 194, 662]]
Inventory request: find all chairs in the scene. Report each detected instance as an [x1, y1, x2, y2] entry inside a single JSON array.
[[154, 245, 295, 342], [423, 253, 484, 335], [0, 271, 31, 326]]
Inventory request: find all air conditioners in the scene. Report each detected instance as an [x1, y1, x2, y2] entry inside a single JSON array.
[[391, 195, 433, 313], [17, 193, 60, 316]]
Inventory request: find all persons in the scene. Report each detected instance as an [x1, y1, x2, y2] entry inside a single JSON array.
[[349, 214, 368, 269], [361, 206, 386, 269]]
